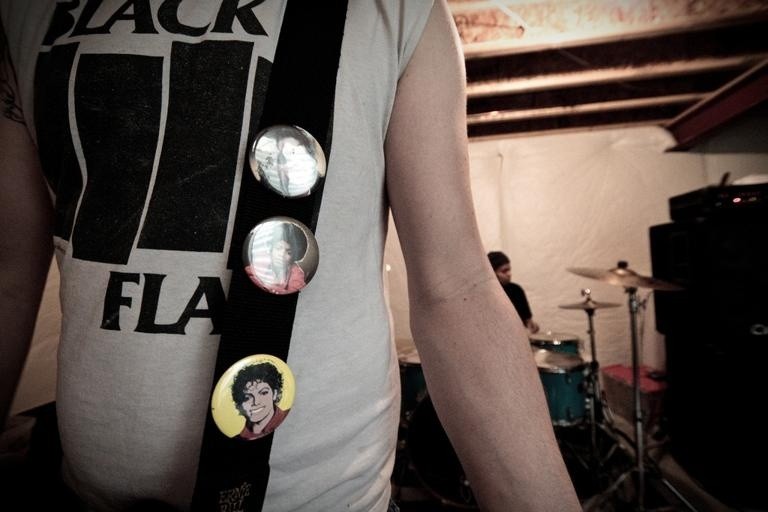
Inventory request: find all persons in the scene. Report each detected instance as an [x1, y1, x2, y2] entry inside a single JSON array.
[[488, 251, 539, 333], [232, 362, 291, 440], [0, 0, 582, 512], [246, 223, 308, 295]]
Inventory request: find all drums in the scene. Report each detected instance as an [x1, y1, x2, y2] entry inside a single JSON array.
[[530, 349, 585, 427], [566, 424, 635, 490], [398, 347, 438, 424], [528, 330, 581, 356]]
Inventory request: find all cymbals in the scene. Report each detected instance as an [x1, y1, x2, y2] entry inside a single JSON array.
[[557, 302, 623, 309], [564, 266, 684, 291]]
